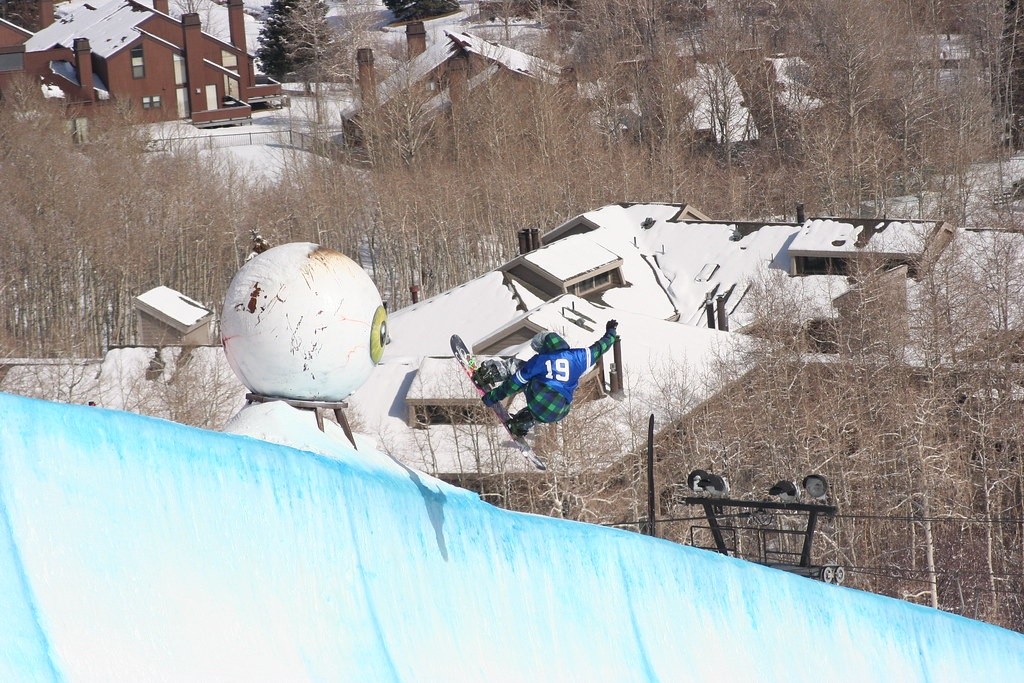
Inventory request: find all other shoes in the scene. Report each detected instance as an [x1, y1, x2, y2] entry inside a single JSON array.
[[472, 360, 497, 387], [506, 413, 530, 441]]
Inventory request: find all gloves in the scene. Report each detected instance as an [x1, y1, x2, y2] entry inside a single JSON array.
[[481, 393, 499, 407], [606, 319, 618, 339]]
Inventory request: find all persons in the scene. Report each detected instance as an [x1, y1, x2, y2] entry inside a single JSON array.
[[472, 319, 619, 438]]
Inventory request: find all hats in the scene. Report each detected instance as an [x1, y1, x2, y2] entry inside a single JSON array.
[[531, 331, 550, 354]]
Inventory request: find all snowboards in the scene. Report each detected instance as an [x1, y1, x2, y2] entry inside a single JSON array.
[[448, 331, 547, 473]]
